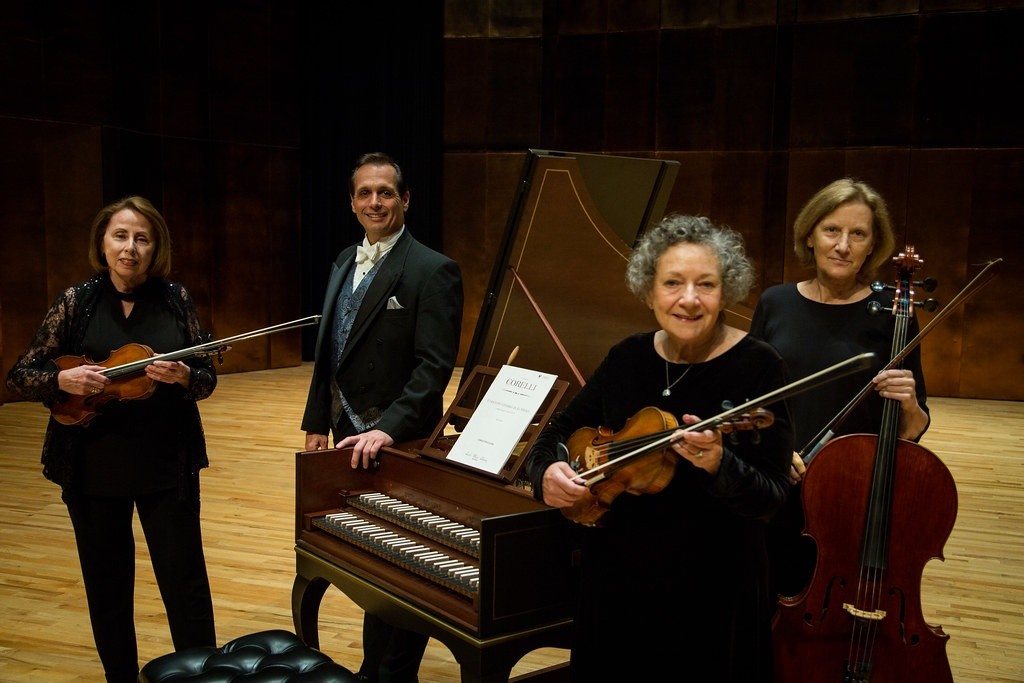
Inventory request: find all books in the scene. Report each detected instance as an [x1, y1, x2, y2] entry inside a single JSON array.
[[446, 365, 558, 476]]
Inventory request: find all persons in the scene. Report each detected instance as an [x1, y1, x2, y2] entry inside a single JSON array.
[[301, 151, 466, 469], [524, 212, 798, 683], [746, 178, 929, 598]]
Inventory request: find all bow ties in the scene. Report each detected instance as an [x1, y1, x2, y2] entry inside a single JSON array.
[[355, 244, 379, 263]]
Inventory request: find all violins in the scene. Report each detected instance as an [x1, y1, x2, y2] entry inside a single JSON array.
[[556, 405, 776, 530], [39, 330, 233, 428]]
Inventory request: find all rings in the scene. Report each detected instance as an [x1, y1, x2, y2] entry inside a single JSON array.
[[92, 387, 96, 392], [7, 196, 219, 683], [694, 450, 703, 457]]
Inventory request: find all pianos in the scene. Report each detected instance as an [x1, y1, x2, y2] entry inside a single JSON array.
[[289, 146, 755, 683]]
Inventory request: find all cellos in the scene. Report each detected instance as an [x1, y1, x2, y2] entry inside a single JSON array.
[[766, 244, 961, 683]]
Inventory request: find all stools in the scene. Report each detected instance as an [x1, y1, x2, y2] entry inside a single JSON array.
[[139, 629, 362, 683]]
